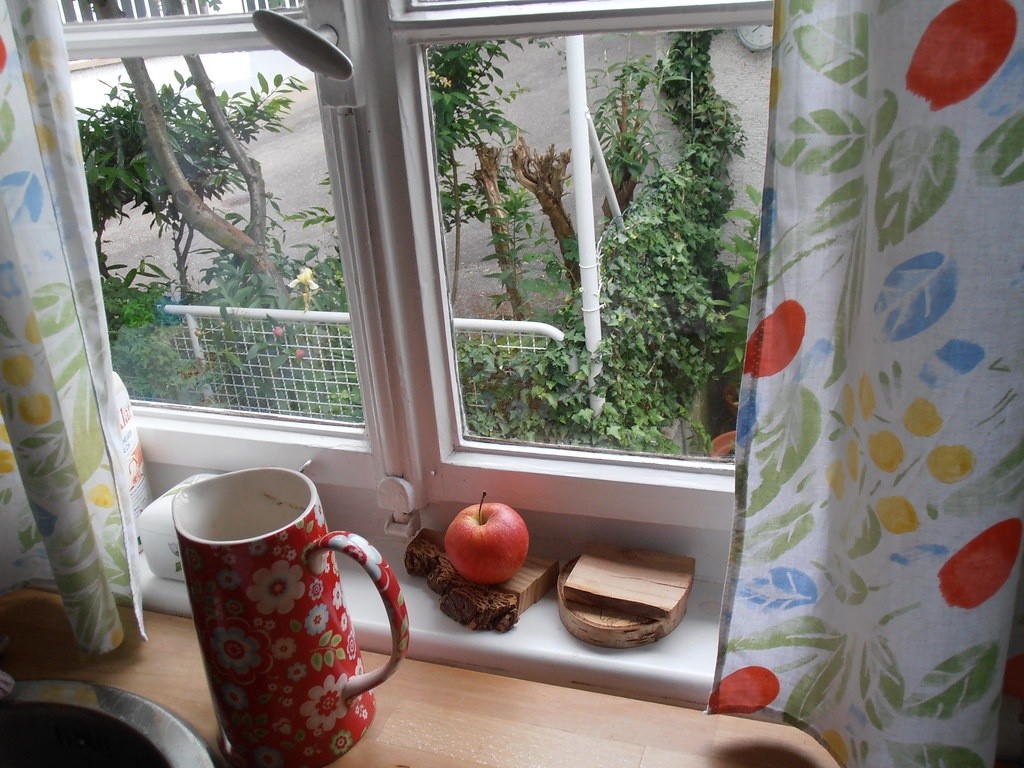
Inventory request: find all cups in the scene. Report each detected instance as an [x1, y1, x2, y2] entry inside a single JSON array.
[[172, 466, 409, 768]]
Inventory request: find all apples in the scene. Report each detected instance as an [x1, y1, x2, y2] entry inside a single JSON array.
[[444, 491, 528, 583]]
